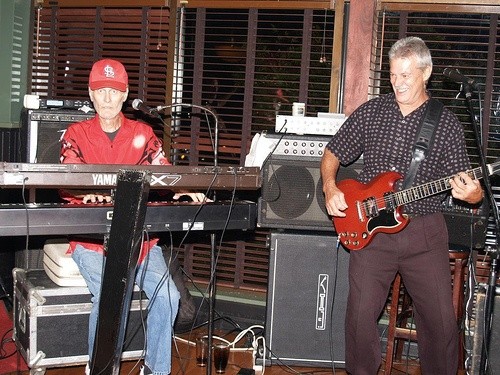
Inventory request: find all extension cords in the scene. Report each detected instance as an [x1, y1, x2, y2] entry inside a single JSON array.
[[252, 349, 263, 371]]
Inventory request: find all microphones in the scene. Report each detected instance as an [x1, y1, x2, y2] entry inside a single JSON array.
[[132, 99, 161, 119], [443, 68, 478, 87]]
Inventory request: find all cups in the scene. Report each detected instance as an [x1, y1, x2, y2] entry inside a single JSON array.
[[214, 342, 230, 374], [195, 335, 210, 367]]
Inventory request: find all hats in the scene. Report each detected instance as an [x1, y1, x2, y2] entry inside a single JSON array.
[[88, 59, 129, 92]]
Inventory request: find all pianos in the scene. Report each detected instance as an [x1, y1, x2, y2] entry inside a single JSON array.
[[0, 162, 259, 375]]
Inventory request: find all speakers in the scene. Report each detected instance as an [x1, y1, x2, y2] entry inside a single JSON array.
[[264, 230, 351, 368], [14, 108, 97, 269], [256, 160, 365, 232], [440, 190, 489, 249]]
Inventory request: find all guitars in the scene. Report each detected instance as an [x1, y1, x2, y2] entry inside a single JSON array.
[[331, 160, 500, 252]]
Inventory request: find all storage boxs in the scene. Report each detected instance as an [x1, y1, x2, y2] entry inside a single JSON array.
[[11, 268, 150, 375]]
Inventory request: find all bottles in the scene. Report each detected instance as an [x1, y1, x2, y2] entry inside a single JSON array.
[[292, 102, 306, 117]]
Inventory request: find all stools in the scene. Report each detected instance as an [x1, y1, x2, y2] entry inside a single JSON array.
[[384, 244, 470, 375]]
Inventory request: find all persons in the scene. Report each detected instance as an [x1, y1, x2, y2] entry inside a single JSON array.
[[59, 59, 213, 375], [320, 37, 484, 375]]
